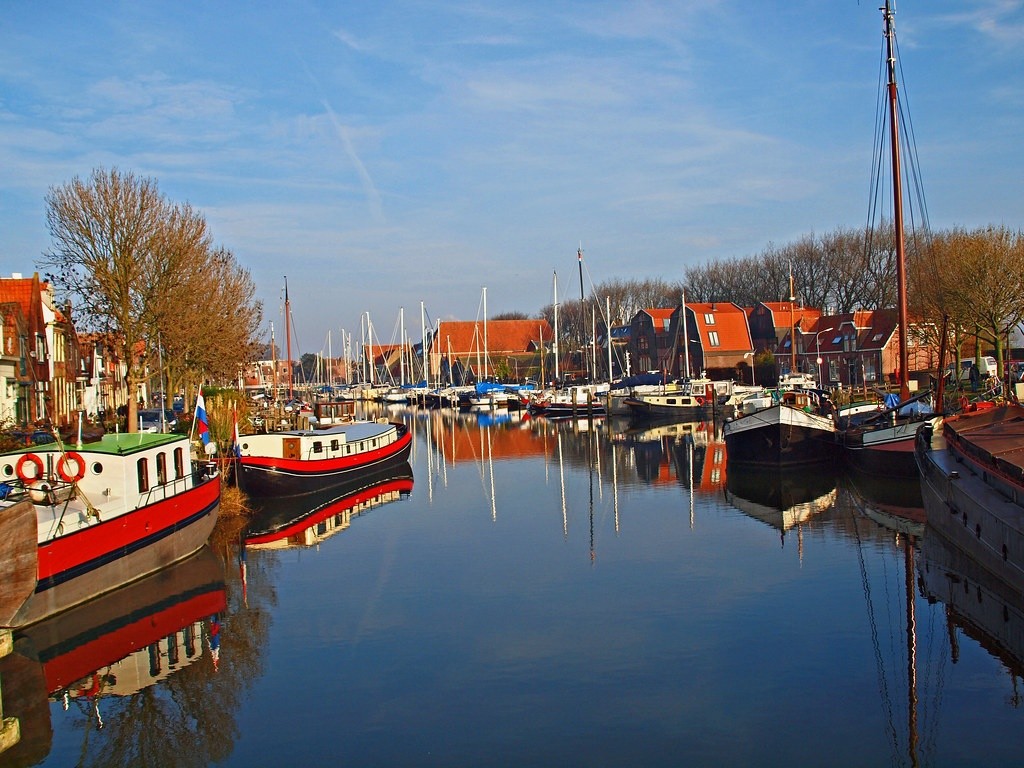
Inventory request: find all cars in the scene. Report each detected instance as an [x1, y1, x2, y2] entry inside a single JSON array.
[[137, 408, 177, 434]]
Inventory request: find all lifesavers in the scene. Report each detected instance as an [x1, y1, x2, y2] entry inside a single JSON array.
[[17, 452, 44, 484], [696, 397, 705, 406], [56, 452, 86, 482], [961, 397, 968, 409], [71, 673, 99, 697]]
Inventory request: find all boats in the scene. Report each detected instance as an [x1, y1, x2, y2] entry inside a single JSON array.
[[723, 463, 836, 549], [238, 417, 413, 498], [244, 460, 413, 551], [913, 400, 1024, 598], [916, 522, 1024, 711], [723, 389, 838, 470], [0, 304, 221, 631], [1, 543, 230, 730]]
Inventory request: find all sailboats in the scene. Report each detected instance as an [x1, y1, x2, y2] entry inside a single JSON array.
[[842, 474, 953, 768], [313, 247, 832, 415], [838, 0, 948, 481], [624, 414, 714, 530]]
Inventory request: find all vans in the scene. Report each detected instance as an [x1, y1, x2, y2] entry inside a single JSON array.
[[947, 356, 997, 380]]
